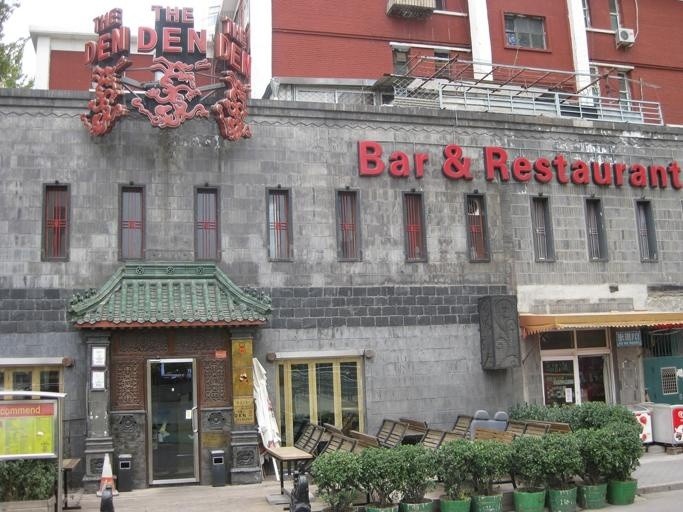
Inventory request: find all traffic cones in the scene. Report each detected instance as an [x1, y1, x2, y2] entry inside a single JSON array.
[[94, 453, 119, 498]]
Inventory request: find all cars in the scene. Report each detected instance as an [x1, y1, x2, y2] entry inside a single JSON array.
[[542, 371, 600, 404], [152, 362, 191, 394], [278, 366, 362, 425]]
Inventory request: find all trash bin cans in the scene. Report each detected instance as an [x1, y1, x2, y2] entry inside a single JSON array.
[[117, 454, 134, 492], [209, 450, 226, 487]]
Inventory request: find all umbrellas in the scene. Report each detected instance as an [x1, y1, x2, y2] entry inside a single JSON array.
[[252, 359, 284, 481]]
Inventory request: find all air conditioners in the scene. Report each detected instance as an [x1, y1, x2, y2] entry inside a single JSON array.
[[615, 28, 636, 49]]
